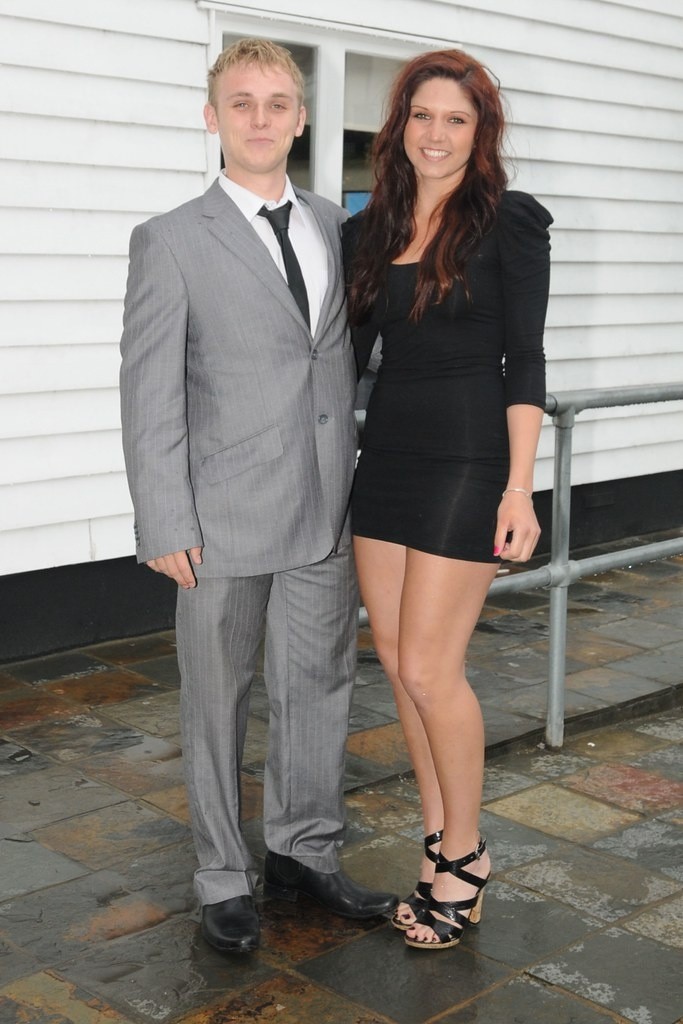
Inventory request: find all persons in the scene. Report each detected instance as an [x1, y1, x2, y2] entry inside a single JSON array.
[[120, 41, 382, 951], [339, 49, 554, 946]]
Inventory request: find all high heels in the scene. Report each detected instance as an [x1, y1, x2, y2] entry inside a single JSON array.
[[405, 837, 491, 949], [390, 829, 443, 930]]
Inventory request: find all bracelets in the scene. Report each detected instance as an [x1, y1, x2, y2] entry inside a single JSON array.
[[502, 489, 531, 497]]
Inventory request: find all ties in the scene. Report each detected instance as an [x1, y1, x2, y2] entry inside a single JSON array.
[[257, 200, 310, 331]]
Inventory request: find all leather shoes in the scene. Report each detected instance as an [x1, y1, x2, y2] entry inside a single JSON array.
[[263, 848, 399, 918], [201, 894, 261, 953]]
